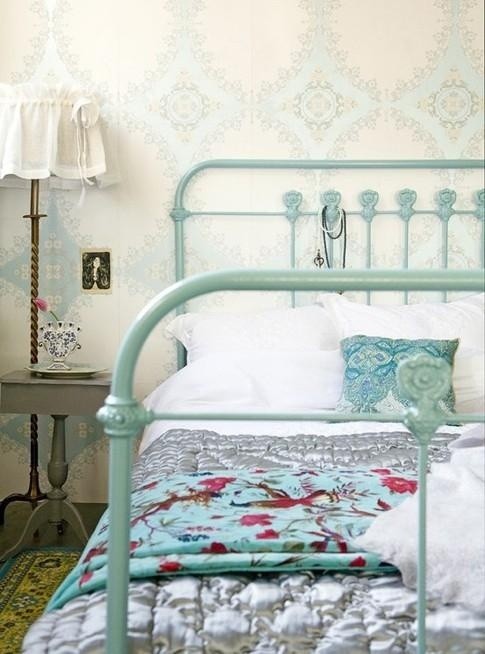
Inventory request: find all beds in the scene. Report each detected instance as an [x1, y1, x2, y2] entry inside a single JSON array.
[[15, 159, 485, 654]]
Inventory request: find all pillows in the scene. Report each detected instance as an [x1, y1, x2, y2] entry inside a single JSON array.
[[142, 291, 485, 426]]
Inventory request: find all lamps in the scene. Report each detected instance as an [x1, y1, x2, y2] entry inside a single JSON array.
[[0, 80, 114, 528]]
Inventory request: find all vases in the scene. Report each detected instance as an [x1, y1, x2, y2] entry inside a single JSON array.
[[35, 320, 82, 369]]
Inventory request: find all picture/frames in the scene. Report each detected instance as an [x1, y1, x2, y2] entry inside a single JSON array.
[[81, 249, 112, 293]]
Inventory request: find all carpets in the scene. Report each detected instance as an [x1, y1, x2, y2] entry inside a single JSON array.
[[0, 544, 87, 654]]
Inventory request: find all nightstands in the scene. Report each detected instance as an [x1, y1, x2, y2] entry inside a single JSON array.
[[0, 369, 113, 562]]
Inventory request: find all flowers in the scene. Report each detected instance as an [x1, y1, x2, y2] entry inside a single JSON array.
[[30, 297, 73, 323]]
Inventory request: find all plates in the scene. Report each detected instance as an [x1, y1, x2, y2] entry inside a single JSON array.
[[24, 359, 115, 377]]
